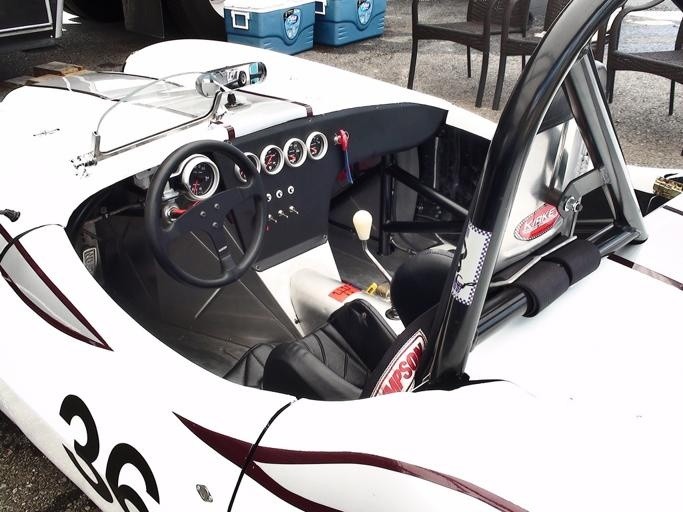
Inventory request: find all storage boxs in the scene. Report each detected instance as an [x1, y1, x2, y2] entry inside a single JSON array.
[[223, 0, 386, 55]]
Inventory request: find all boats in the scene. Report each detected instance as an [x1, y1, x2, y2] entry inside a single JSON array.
[[0, 0, 683, 512]]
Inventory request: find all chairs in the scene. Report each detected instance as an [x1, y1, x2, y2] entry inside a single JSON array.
[[406, 0, 683, 115], [252, 298, 451, 398]]
